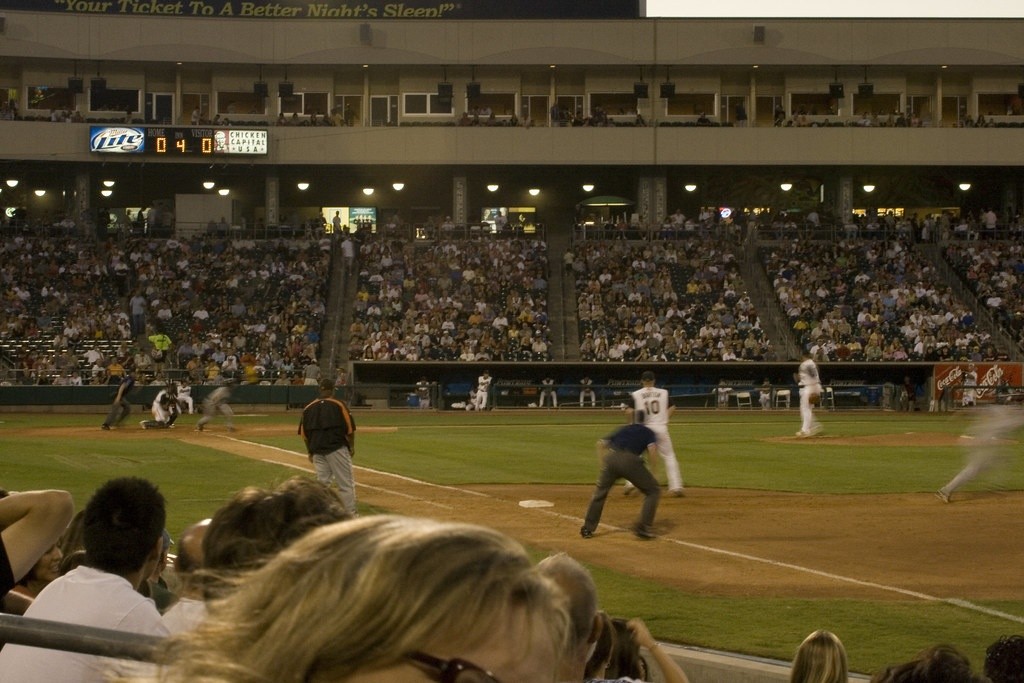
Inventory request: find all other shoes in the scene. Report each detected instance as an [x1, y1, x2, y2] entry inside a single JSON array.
[[189, 411, 194, 414], [140, 421, 147, 430], [936, 490, 951, 504], [196, 423, 203, 431], [101, 424, 111, 430], [165, 422, 175, 428], [625, 489, 640, 496], [673, 489, 685, 497], [579, 527, 593, 539], [635, 527, 654, 539]]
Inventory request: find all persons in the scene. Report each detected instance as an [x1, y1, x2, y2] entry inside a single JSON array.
[[474, 370, 493, 411], [959, 363, 977, 407], [579, 409, 661, 540], [795, 344, 825, 437], [298, 379, 359, 517], [758, 378, 774, 410], [577, 376, 598, 407], [620, 371, 689, 497], [537, 378, 559, 409], [100, 370, 144, 432], [0, 104, 1024, 683], [713, 379, 733, 409]]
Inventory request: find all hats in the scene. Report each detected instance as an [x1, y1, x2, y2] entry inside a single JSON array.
[[483, 369, 488, 373], [161, 528, 175, 549], [319, 379, 334, 391], [641, 371, 655, 382]]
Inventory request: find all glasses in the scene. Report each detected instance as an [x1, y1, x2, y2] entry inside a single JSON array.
[[404, 650, 499, 683]]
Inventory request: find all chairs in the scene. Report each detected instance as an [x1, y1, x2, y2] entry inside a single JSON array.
[[0, 115, 961, 411]]
